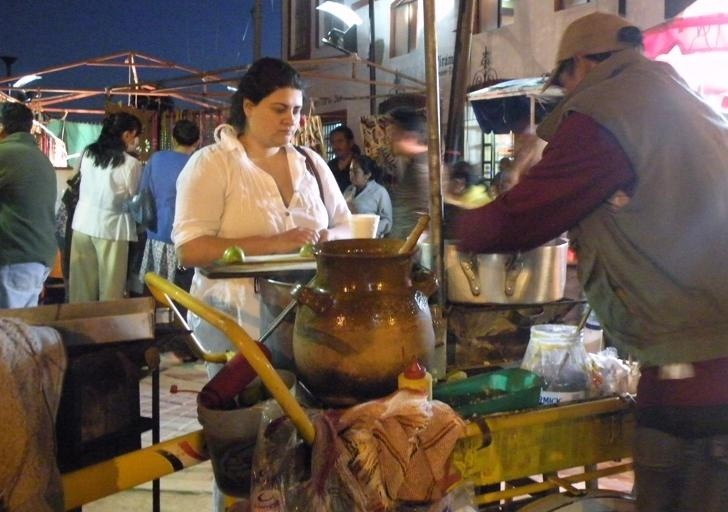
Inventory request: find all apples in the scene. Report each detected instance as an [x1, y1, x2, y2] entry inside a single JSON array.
[[300, 244, 314, 257], [223, 248, 244, 262]]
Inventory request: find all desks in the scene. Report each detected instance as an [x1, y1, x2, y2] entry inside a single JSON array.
[[0, 297, 165, 512]]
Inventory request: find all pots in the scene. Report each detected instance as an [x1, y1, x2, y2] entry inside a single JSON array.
[[404, 229, 571, 309], [289, 235, 439, 412], [252, 273, 317, 373]]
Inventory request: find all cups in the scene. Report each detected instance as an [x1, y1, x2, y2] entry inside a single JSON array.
[[348, 213, 383, 239]]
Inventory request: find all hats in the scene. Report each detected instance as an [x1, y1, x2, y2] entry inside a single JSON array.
[[536, 12, 645, 96]]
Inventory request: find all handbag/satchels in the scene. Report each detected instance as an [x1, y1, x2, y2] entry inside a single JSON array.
[[127, 186, 155, 227], [62, 173, 82, 210]]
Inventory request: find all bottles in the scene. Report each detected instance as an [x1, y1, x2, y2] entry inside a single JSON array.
[[518, 321, 595, 408], [397, 356, 434, 404], [583, 320, 605, 354]]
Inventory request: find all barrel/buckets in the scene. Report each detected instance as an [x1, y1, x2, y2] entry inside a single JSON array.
[[196, 369, 297, 498]]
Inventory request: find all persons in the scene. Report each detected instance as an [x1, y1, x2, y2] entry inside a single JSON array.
[[446, 12, 728, 511], [68, 112, 145, 303], [444, 155, 516, 198], [1, 102, 58, 310], [126, 120, 203, 296], [327, 126, 361, 192], [385, 107, 433, 277], [344, 155, 394, 240], [171, 57, 355, 382]]
